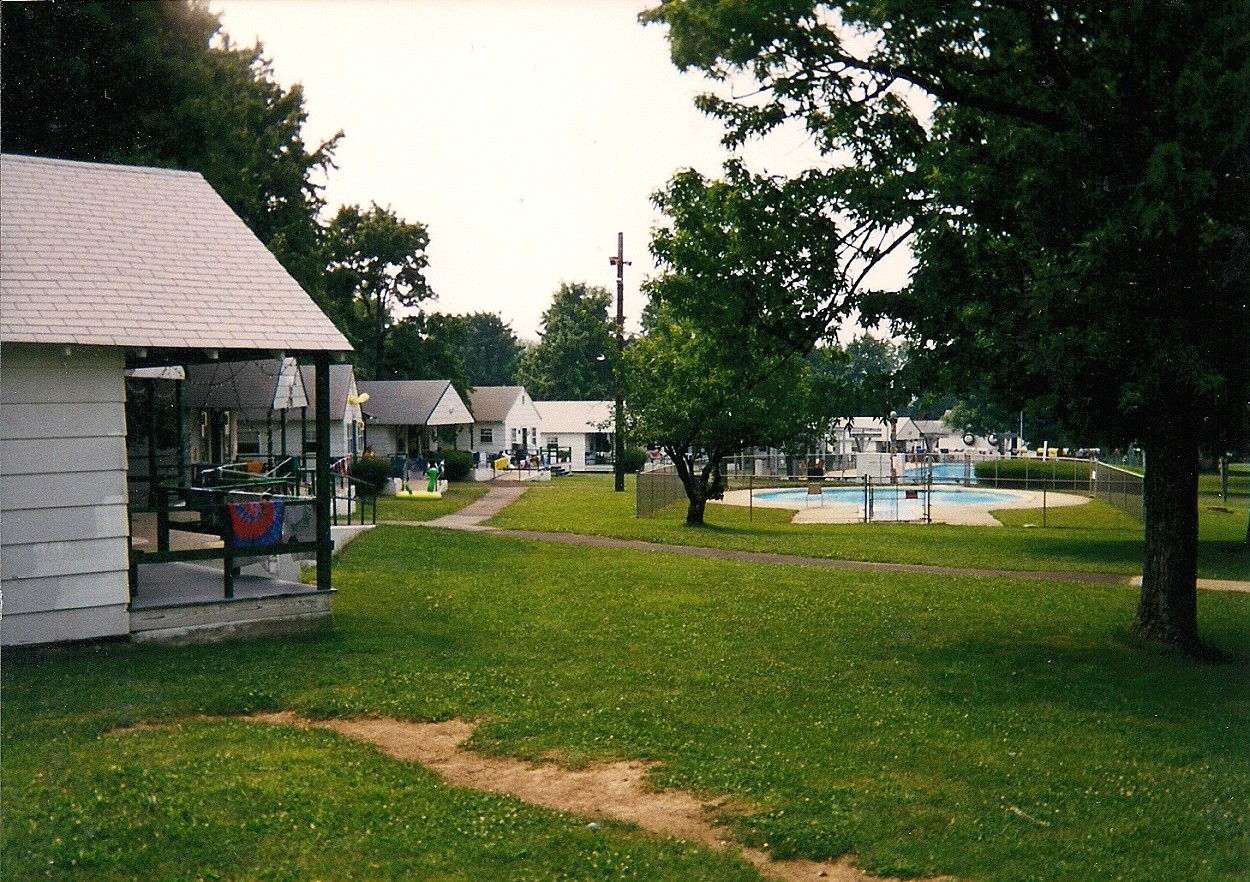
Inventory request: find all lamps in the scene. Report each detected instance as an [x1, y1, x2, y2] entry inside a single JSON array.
[[349, 393, 370, 405]]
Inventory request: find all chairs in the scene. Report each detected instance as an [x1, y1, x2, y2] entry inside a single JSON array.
[[806, 483, 824, 508]]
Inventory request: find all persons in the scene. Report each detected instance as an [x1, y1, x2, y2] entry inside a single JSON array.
[[653, 447, 661, 458], [495, 451, 503, 459], [818, 455, 824, 469], [364, 445, 374, 458]]
